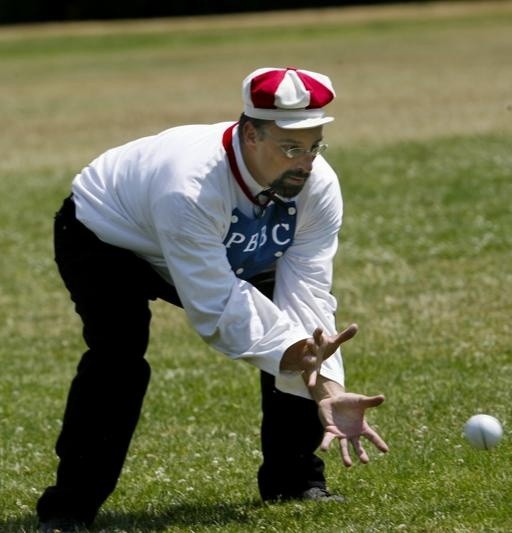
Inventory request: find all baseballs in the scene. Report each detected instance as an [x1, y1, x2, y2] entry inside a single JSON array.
[[464, 414, 503, 450]]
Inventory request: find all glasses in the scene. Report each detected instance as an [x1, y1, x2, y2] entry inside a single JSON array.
[[263, 133, 329, 159]]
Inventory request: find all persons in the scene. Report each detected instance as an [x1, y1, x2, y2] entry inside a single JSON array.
[[38, 65, 388, 532]]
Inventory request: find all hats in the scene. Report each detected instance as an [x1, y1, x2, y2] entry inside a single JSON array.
[[241, 64, 336, 129]]
